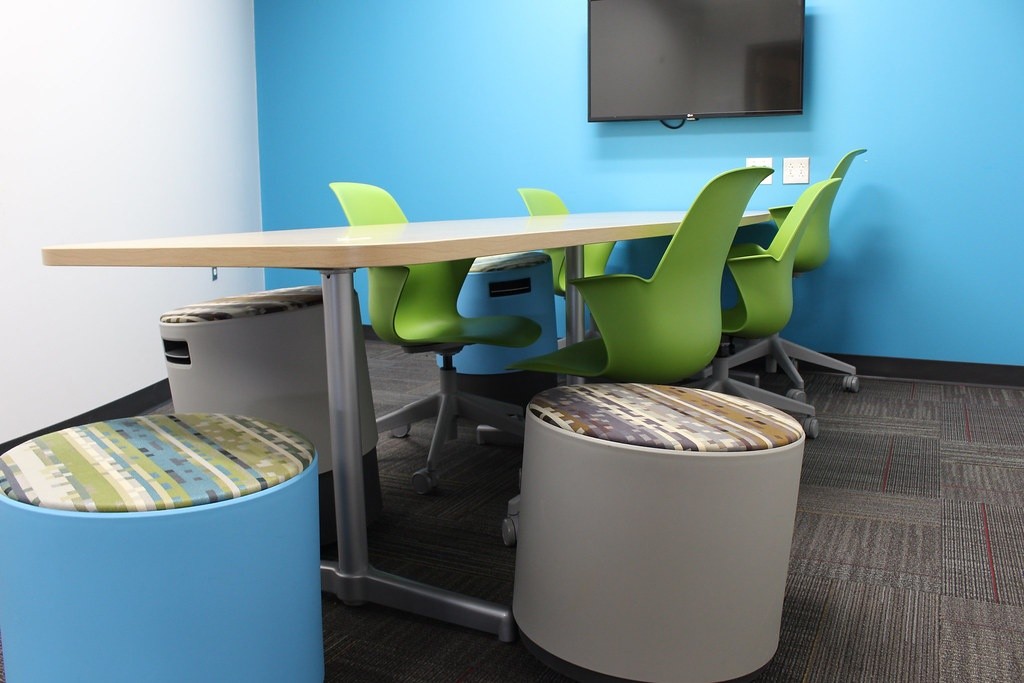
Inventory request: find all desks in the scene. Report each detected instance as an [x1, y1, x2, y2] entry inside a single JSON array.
[[40, 210, 773, 642]]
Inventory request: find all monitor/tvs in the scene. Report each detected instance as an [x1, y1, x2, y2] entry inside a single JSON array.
[[588, 0, 804, 122]]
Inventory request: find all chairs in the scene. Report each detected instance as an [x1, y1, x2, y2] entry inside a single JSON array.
[[329, 149, 867, 547]]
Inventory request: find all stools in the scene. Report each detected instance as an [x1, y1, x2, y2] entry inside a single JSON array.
[[0, 413, 323, 683], [512, 384, 805, 683], [160, 285, 378, 474], [435, 251, 558, 374]]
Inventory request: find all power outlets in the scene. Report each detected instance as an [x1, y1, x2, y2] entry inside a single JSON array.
[[783, 157, 809, 184], [746, 157, 773, 185]]
